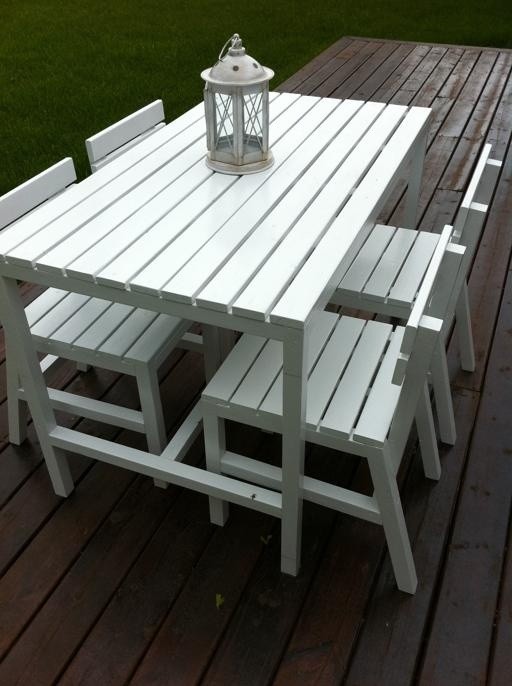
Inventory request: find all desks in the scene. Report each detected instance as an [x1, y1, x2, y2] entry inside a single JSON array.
[[1, 86, 431, 577]]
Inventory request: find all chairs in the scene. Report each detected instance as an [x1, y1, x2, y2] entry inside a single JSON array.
[[0, 153, 199, 498], [199, 225, 483, 588], [286, 137, 504, 442], [84, 97, 167, 174]]
